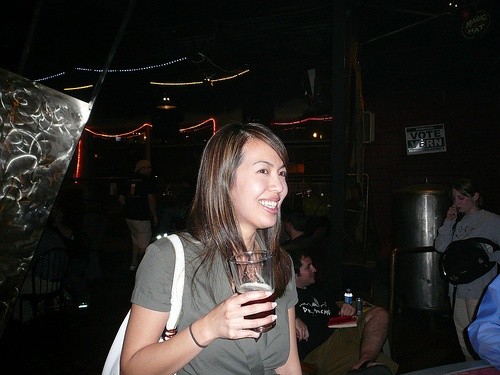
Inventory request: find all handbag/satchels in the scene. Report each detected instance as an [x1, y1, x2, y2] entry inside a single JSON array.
[[439, 236, 500, 284], [102, 234, 186, 375]]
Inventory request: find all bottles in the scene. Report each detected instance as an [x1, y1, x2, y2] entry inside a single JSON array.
[[344, 289, 353, 304], [355, 298, 363, 315]]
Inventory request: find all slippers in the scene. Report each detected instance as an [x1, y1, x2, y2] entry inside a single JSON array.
[[349, 360, 393, 375]]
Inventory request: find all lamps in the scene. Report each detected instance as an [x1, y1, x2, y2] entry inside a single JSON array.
[[154, 84, 176, 111]]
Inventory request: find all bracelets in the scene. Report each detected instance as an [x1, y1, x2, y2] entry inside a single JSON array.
[[189, 323, 207, 348]]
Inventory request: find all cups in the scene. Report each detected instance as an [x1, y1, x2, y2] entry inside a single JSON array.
[[229, 251, 276, 332]]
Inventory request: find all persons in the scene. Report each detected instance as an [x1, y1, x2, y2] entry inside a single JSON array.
[[120, 121, 301, 375], [280, 174, 500, 375], [174, 174, 196, 218], [50, 159, 159, 311]]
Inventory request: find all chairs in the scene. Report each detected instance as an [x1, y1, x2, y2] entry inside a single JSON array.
[[18, 248, 73, 318]]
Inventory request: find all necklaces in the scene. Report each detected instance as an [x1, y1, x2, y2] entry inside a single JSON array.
[[232, 241, 261, 283]]
